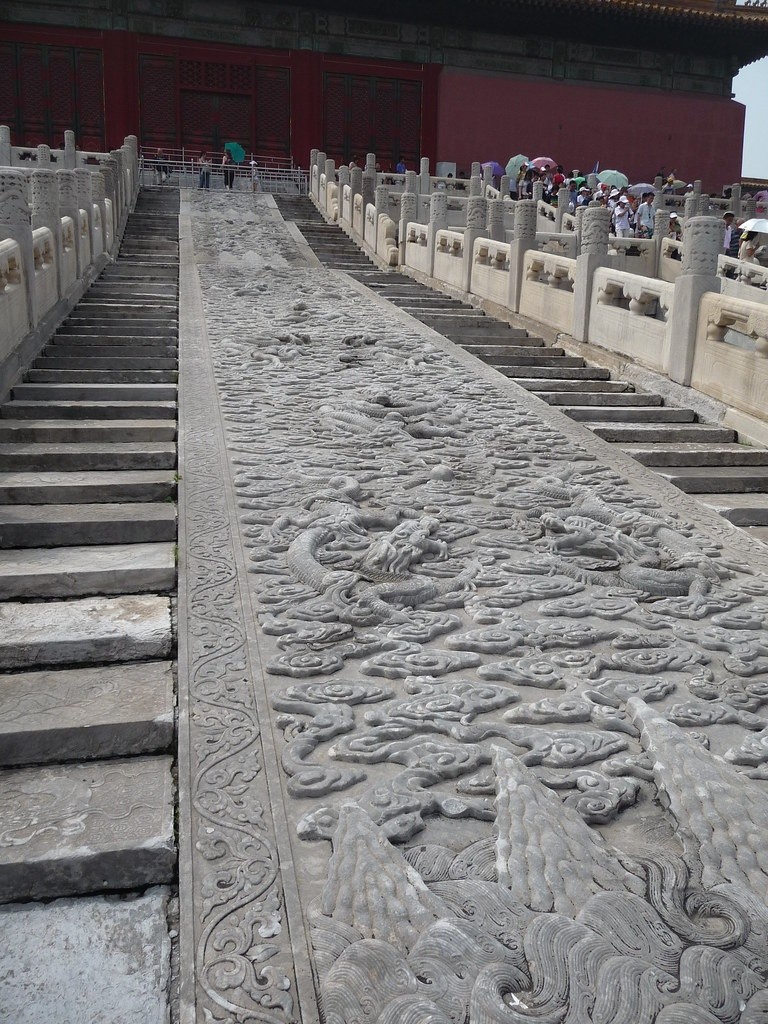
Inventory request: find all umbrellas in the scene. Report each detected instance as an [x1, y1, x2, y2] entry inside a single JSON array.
[[224, 142, 246, 163], [662, 180, 687, 189], [738, 218, 767, 233], [480, 154, 656, 195]]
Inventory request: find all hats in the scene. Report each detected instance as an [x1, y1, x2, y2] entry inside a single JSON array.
[[250, 160, 259, 166], [515, 159, 734, 224], [352, 155, 361, 160]]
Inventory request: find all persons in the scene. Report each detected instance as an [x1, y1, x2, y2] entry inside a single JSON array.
[[445, 164, 768, 282], [396, 156, 405, 174], [348, 157, 359, 169], [376, 162, 384, 172], [220, 149, 234, 190], [198, 150, 211, 188], [153, 147, 167, 186]]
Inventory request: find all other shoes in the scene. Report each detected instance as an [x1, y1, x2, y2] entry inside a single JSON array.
[[153, 178, 236, 190]]
